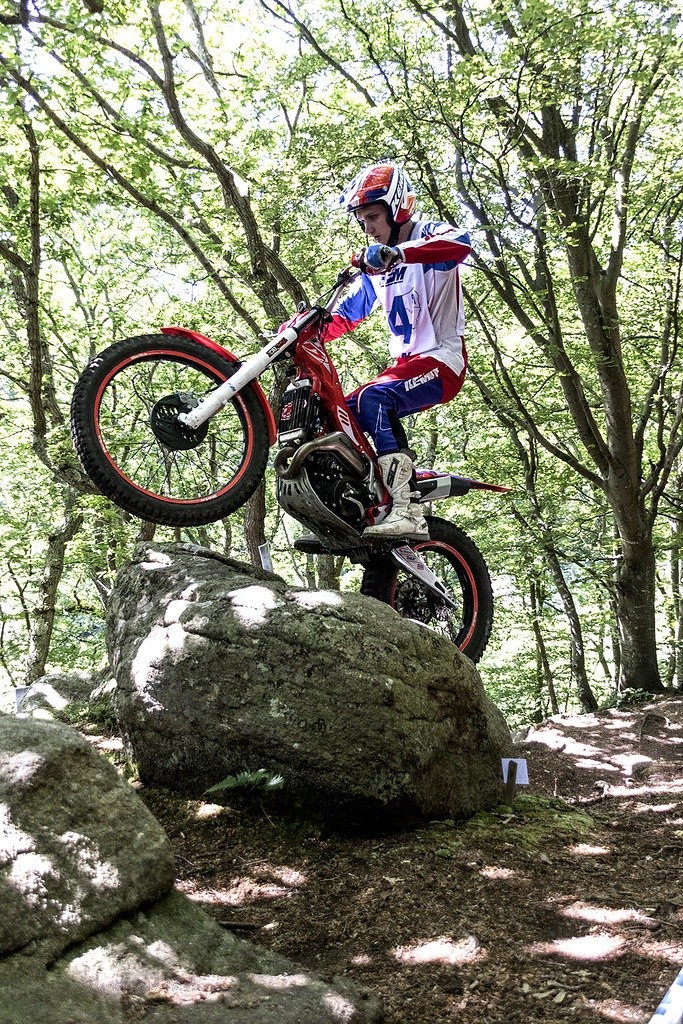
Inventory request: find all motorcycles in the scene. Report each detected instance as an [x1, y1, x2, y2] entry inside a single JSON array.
[[68, 244, 516, 669]]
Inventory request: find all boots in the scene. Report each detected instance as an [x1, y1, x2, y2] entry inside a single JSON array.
[[362, 452, 430, 541], [294, 534, 329, 554]]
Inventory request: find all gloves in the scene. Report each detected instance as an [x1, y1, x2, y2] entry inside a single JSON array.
[[358, 243, 403, 276]]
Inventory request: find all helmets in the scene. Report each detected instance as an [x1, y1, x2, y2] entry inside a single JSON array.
[[339, 164, 416, 229]]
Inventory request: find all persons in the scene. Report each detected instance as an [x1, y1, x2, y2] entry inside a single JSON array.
[[280, 162, 472, 555]]
